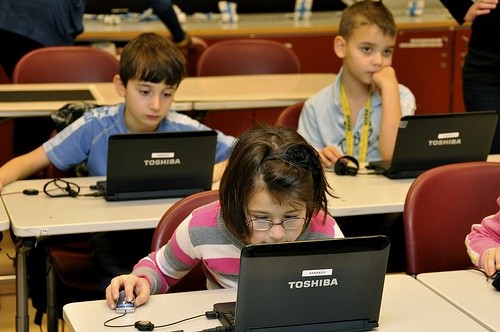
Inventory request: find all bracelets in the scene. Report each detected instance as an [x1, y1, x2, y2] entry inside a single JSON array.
[[177, 35, 192, 49]]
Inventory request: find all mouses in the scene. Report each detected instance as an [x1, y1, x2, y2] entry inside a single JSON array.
[[114, 289, 136, 313]]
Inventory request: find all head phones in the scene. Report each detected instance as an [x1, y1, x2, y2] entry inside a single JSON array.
[[334, 155, 360, 176]]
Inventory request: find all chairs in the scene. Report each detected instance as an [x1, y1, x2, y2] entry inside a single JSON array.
[[404, 161, 500, 275], [197, 39, 303, 75], [12, 47, 119, 81]]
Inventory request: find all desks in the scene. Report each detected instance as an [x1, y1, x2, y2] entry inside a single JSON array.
[[0, 73, 338, 179], [0, 155, 500, 332], [75, 0, 458, 139]]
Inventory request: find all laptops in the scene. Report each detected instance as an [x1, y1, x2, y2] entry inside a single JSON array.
[[369, 111, 498, 179], [213, 234, 390, 332], [97, 130, 217, 201]]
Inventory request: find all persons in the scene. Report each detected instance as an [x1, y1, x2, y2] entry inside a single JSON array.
[[465, 195, 500, 278], [438, 0, 500, 155], [297, 0, 417, 274], [0, 0, 208, 246], [106, 122, 346, 310], [0, 32, 238, 294]]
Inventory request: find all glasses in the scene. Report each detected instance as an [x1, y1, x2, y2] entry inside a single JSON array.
[[245, 218, 307, 231], [44, 179, 80, 198]]
